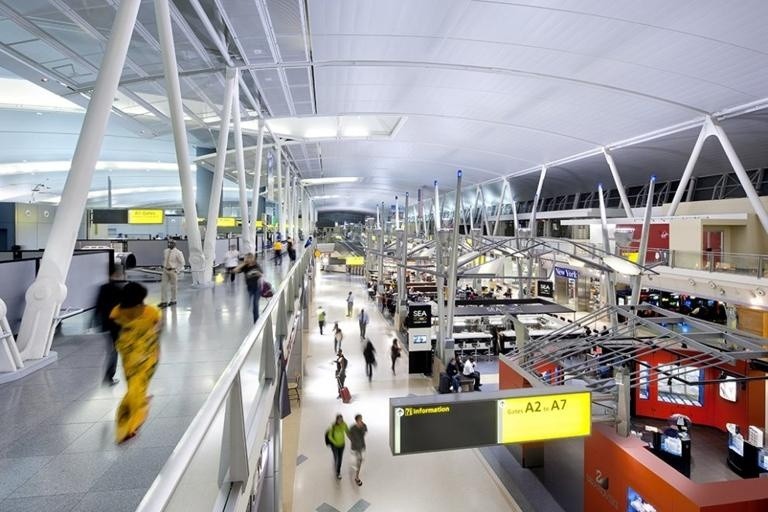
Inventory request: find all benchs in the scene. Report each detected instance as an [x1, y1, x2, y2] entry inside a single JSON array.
[[455, 372, 473, 392]]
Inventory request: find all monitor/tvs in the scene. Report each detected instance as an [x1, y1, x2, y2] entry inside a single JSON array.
[[537, 281, 553, 297]]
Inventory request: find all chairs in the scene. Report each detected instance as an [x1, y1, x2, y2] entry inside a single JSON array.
[[456, 336, 495, 364], [287, 375, 301, 409]]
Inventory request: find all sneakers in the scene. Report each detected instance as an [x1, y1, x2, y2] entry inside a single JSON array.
[[336, 473, 342, 479], [168, 302, 176, 305], [158, 303, 167, 307], [355, 478, 362, 486]]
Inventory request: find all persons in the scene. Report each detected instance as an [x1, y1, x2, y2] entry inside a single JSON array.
[[158, 239, 185, 308], [223, 236, 312, 324], [328, 414, 351, 479], [349, 415, 367, 486], [316, 280, 511, 399], [95, 264, 130, 387], [109, 281, 163, 445]]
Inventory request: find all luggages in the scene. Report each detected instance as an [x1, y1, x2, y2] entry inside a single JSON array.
[[440, 376, 450, 393], [337, 376, 351, 402]]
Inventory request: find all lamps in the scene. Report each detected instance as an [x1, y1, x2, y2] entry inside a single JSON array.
[[651, 340, 728, 387]]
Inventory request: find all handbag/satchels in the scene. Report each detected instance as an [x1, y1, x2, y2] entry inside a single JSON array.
[[261, 282, 273, 297], [325, 431, 331, 445]]
